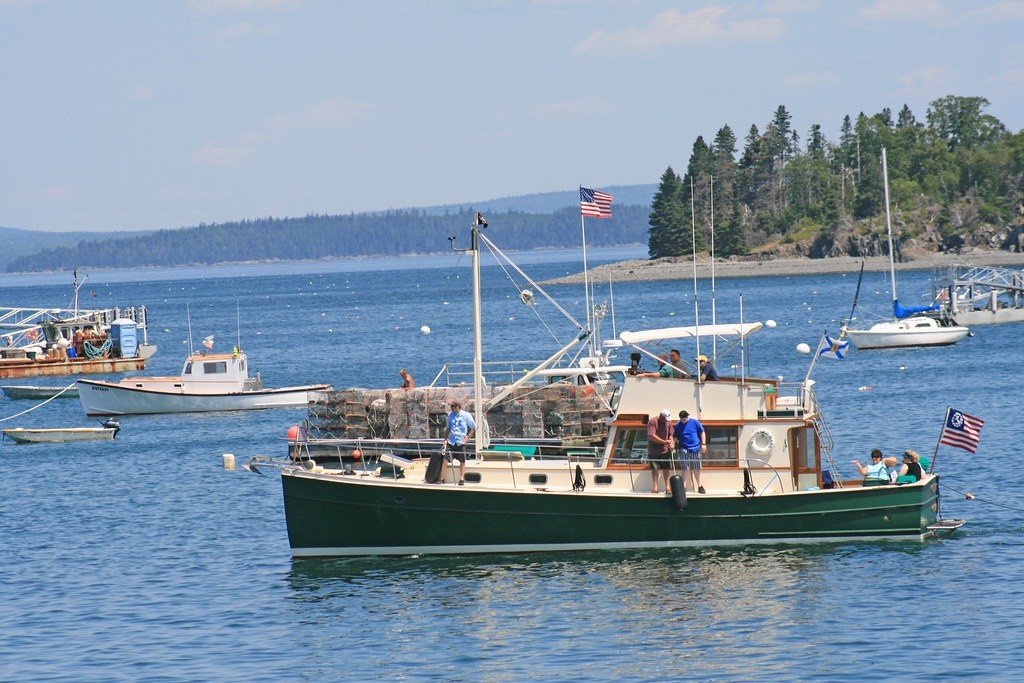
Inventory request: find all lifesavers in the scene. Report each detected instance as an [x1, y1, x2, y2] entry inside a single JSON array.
[[749, 429, 775, 455], [28, 329, 38, 339]]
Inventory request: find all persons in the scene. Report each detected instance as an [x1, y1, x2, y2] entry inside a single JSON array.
[[898, 450, 926, 480], [672, 410, 708, 494], [852, 449, 897, 479], [399, 369, 414, 390], [636, 349, 673, 378], [670, 349, 692, 379], [647, 409, 675, 494], [695, 354, 719, 381], [436, 401, 478, 486]]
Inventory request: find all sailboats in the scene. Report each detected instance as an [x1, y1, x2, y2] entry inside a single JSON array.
[[839, 150, 969, 351]]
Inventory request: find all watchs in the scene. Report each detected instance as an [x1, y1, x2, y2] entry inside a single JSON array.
[[468, 434, 472, 438]]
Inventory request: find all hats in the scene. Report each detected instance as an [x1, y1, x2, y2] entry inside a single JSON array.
[[694, 355, 708, 362], [901, 453, 910, 458], [660, 410, 672, 421], [679, 410, 690, 418]]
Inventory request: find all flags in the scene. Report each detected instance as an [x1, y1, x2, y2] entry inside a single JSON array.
[[818, 335, 849, 360], [479, 212, 488, 229], [580, 187, 615, 219], [939, 408, 984, 454], [91, 288, 97, 297]]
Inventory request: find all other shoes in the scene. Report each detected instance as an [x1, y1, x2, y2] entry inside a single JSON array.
[[458, 479, 464, 486], [698, 486, 705, 493], [437, 480, 445, 484]]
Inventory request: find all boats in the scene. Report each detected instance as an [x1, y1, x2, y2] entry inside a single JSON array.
[[240, 173, 966, 560], [76, 300, 335, 418], [927, 259, 1024, 327], [0, 266, 158, 380], [2, 417, 122, 444], [1, 384, 79, 398]]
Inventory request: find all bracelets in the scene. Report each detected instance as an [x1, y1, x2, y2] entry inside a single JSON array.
[[701, 443, 706, 445]]
